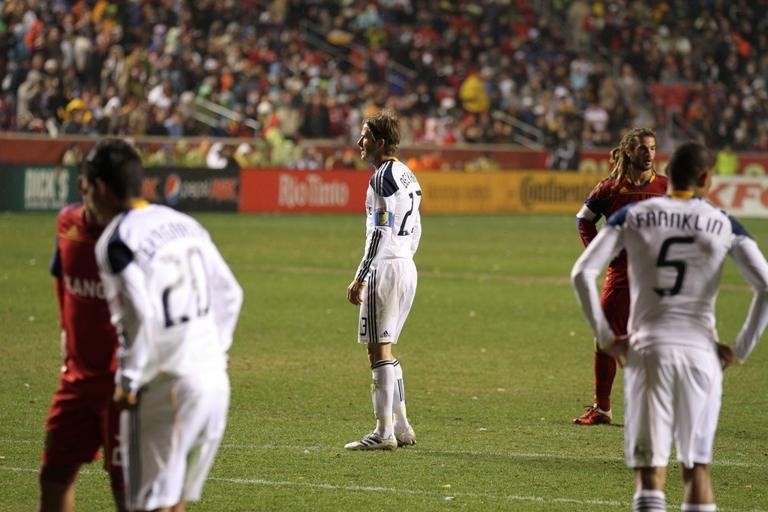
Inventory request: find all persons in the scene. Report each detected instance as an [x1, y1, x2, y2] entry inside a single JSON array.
[[573, 128, 671, 429], [78, 130, 244, 511], [340, 114, 425, 451], [36, 165, 132, 512], [1, 0, 767, 174], [563, 135, 768, 510]]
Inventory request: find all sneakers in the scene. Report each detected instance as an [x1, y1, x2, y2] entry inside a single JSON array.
[[394, 429, 418, 447], [574, 405, 613, 426], [344, 433, 398, 452]]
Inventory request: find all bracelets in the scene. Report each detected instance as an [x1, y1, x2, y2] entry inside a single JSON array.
[[354, 280, 367, 287]]
